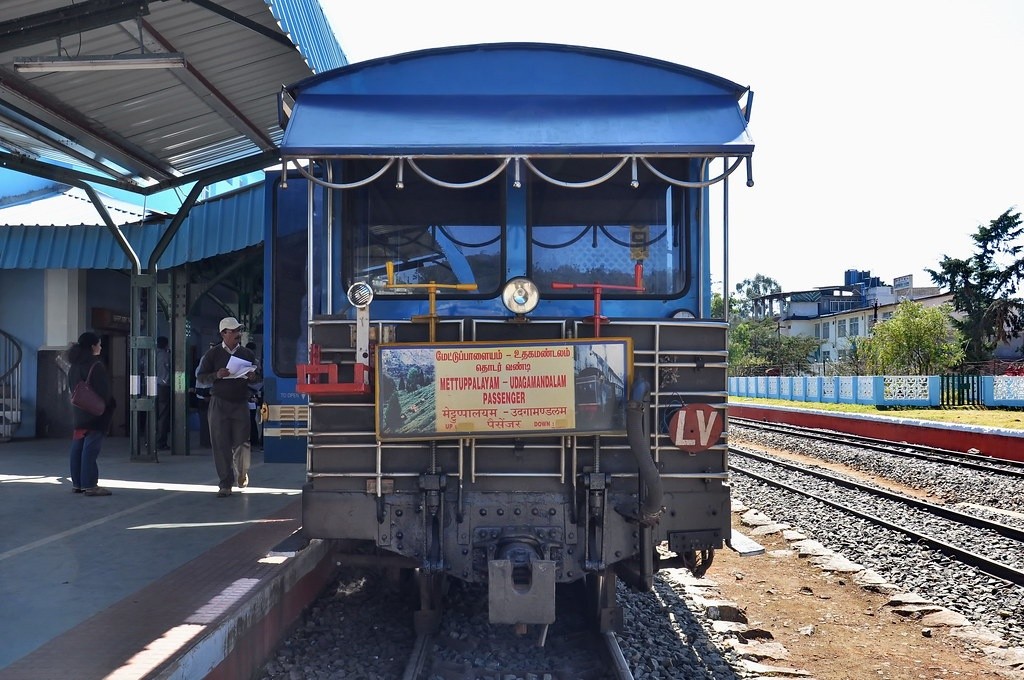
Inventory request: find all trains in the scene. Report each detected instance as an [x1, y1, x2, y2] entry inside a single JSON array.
[[250, 39, 761, 636]]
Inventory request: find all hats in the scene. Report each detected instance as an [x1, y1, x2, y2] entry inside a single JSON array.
[[219, 316, 244, 332]]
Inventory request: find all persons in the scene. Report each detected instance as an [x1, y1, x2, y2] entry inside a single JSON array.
[[64, 330, 116, 496], [244, 343, 260, 445], [196, 317, 258, 498], [139, 336, 172, 451], [188, 377, 212, 449]]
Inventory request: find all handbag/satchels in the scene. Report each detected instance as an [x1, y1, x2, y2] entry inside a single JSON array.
[[71, 363, 106, 416]]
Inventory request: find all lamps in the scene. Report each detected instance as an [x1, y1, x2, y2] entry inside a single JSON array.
[[13, 17, 187, 74]]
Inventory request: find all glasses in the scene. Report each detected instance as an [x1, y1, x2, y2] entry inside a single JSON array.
[[225, 329, 242, 334]]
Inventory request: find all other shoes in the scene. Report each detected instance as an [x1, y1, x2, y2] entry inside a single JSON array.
[[84, 486, 113, 496], [217, 486, 232, 495], [71, 487, 86, 493], [158, 444, 170, 450], [238, 472, 249, 487]]
